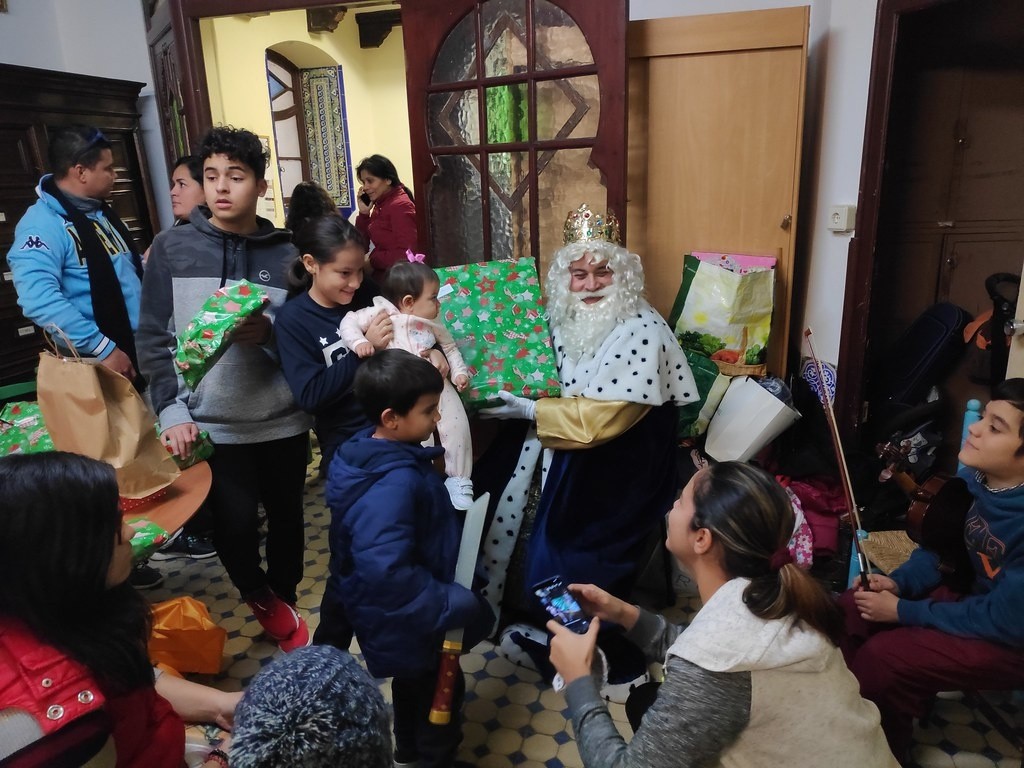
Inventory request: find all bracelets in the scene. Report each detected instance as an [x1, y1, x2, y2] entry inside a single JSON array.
[[205, 749, 229, 768]]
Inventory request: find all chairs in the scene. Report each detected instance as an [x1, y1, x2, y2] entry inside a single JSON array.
[[847, 399, 984, 591]]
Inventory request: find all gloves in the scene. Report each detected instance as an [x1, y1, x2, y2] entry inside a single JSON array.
[[478, 391, 538, 419]]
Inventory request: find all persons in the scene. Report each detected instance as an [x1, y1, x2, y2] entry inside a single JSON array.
[[272, 218, 451, 679], [0, 449, 247, 768], [835, 378, 1024, 763], [546, 461, 901, 768], [326, 348, 497, 768], [5, 122, 216, 591], [137, 125, 313, 656], [233, 644, 399, 768], [348, 155, 418, 294], [141, 154, 273, 547], [478, 203, 698, 703], [286, 178, 358, 286], [339, 248, 476, 511]]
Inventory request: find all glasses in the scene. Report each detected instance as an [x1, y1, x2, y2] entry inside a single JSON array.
[[82, 126, 107, 155]]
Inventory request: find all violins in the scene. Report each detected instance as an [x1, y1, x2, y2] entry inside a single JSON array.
[[876, 440, 973, 592]]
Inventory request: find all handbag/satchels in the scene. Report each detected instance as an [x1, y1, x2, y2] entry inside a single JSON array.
[[36, 323, 182, 500], [663, 253, 803, 463]]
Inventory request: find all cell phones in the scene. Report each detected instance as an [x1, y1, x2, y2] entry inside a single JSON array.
[[533, 574, 591, 634]]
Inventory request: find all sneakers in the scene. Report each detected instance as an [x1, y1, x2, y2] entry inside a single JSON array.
[[444, 476, 474, 511], [124, 560, 163, 590], [147, 528, 217, 560], [273, 601, 310, 654], [245, 585, 299, 641]]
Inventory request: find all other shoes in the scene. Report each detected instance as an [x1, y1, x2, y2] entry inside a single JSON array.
[[390, 749, 418, 767]]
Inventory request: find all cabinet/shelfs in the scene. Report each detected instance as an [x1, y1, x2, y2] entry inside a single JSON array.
[[0, 63, 166, 387]]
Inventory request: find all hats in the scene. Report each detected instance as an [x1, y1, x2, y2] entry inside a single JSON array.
[[230, 646, 395, 768]]
